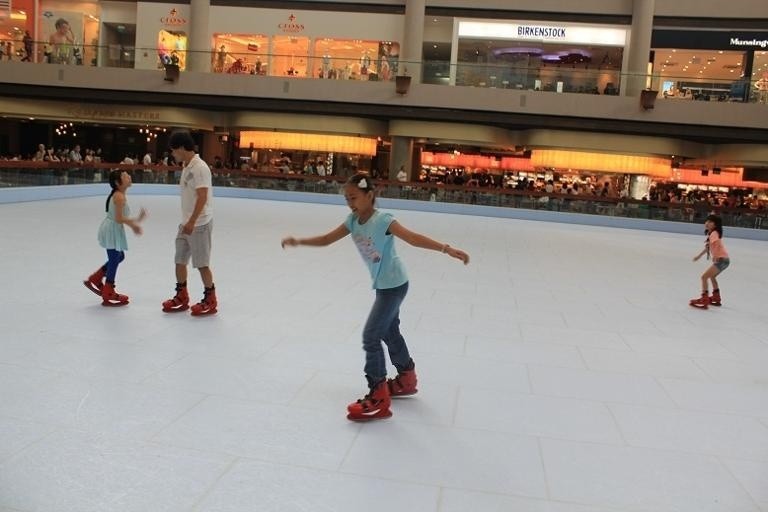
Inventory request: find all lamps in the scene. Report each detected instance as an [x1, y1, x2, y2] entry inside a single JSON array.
[[395, 75, 412, 94], [638, 87, 660, 112], [217, 135, 229, 143], [162, 62, 180, 81]]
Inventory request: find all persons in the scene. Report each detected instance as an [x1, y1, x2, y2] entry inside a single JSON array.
[[210, 156, 327, 192], [255, 54, 262, 73], [320, 48, 332, 80], [396, 165, 609, 216], [79, 167, 149, 301], [684, 214, 733, 305], [6, 42, 13, 62], [161, 129, 218, 313], [34, 144, 105, 184], [636, 183, 768, 229], [47, 17, 75, 48], [359, 51, 371, 80], [19, 30, 34, 63], [217, 45, 229, 72], [279, 169, 468, 414], [159, 37, 171, 66], [379, 46, 396, 81], [123, 151, 182, 185], [0, 41, 7, 61], [615, 184, 629, 209]]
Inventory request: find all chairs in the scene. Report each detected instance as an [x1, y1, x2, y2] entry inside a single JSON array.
[[447, 183, 768, 236], [173, 172, 447, 202]]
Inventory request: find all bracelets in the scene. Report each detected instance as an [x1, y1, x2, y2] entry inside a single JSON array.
[[440, 242, 451, 256]]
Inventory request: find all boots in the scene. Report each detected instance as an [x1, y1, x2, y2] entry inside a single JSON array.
[[348, 380, 391, 416], [710, 289, 721, 303], [163, 280, 189, 308], [690, 291, 709, 304], [388, 370, 418, 394], [89, 270, 106, 290], [191, 284, 217, 313], [102, 283, 128, 300]]
